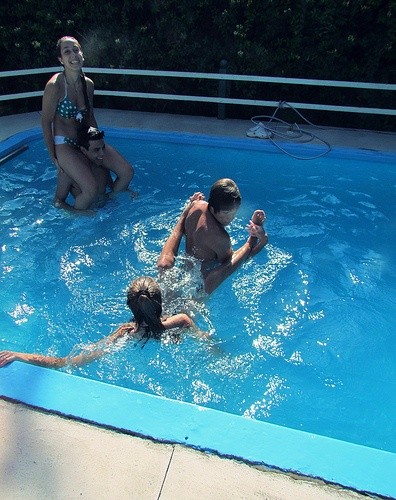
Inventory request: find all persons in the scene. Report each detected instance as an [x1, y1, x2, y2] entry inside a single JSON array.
[[41, 36, 134, 200], [54, 126, 115, 209], [184, 178, 269, 262], [0, 192, 267, 367]]
